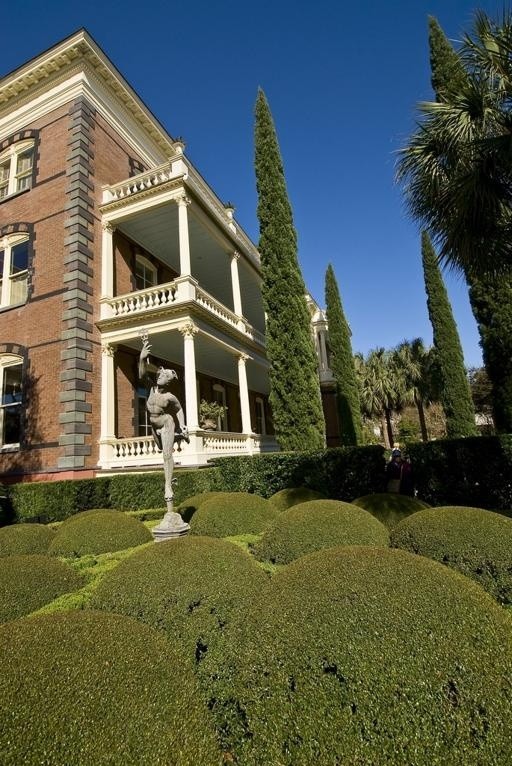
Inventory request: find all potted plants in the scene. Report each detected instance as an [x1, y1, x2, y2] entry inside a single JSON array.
[[200, 400, 228, 430]]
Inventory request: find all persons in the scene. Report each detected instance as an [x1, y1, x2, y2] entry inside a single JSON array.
[[139, 340, 189, 499], [388, 449, 414, 497]]
